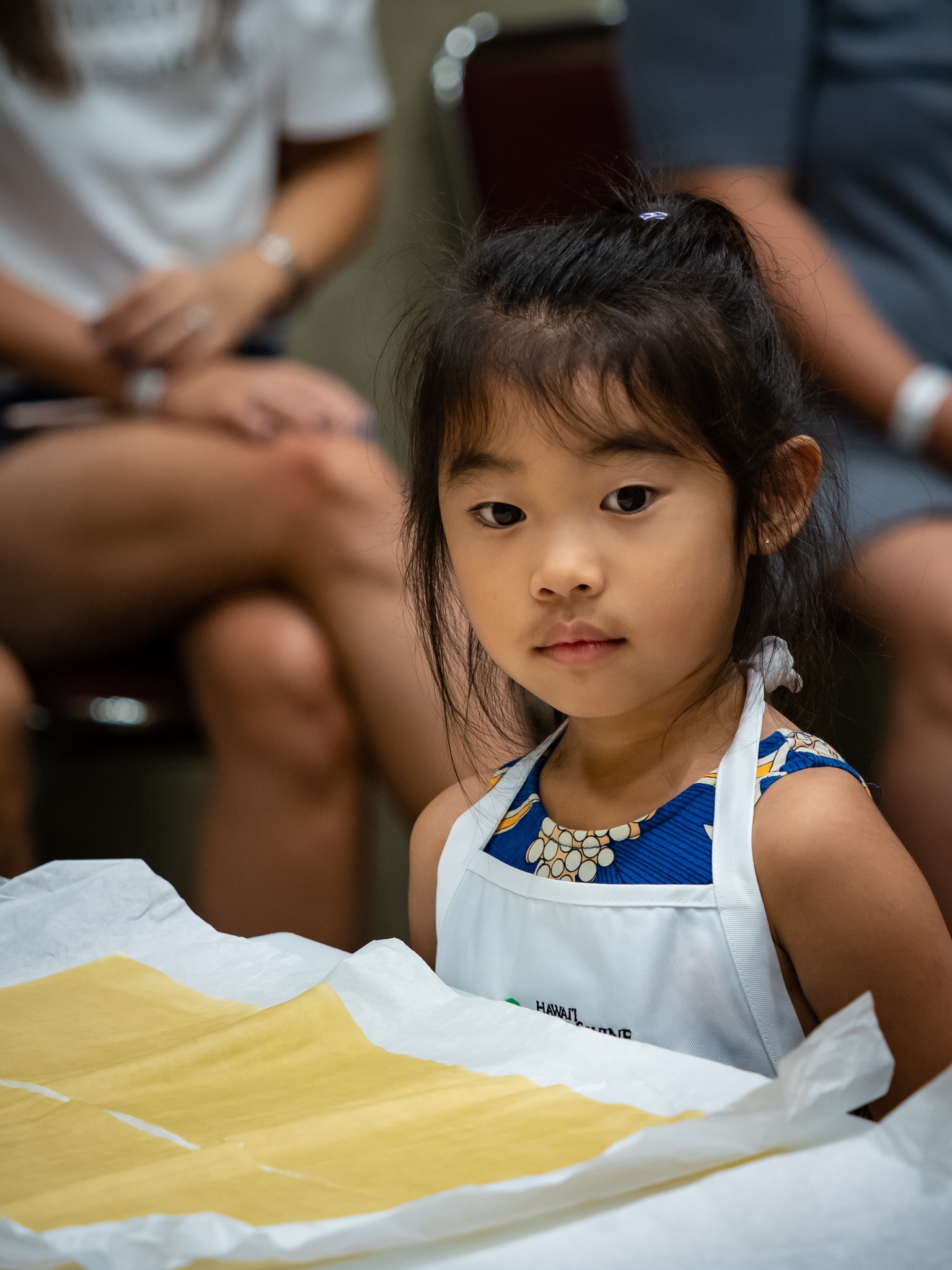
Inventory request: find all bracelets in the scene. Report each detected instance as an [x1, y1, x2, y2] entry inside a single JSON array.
[[124, 362, 166, 415], [249, 228, 313, 316], [890, 363, 947, 455]]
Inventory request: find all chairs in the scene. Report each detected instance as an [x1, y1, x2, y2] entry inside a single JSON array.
[[423, 19, 901, 772]]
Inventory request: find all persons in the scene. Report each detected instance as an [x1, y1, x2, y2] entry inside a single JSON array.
[[2, 2, 537, 957], [606, 1, 949, 932], [402, 186, 951, 1129]]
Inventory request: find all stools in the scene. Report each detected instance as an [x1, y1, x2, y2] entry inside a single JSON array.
[[19, 653, 211, 885]]
